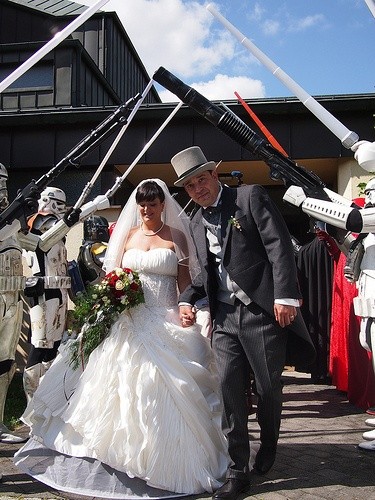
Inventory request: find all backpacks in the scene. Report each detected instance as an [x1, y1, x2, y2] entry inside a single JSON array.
[[67, 260, 88, 301]]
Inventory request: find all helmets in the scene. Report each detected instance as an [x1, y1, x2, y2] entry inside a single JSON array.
[[37, 186, 67, 215], [0, 163, 10, 210], [83, 214, 109, 241]]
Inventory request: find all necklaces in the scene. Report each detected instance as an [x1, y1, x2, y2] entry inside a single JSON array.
[[140, 221, 165, 235]]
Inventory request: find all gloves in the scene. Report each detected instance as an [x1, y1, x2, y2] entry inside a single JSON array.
[[16, 178, 42, 219], [63, 206, 83, 226]]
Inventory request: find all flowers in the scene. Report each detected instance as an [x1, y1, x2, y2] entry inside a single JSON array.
[[226, 215, 245, 233], [352, 182, 369, 208], [63, 268, 146, 372]]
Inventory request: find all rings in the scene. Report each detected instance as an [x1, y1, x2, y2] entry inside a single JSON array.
[[290, 316, 294, 318]]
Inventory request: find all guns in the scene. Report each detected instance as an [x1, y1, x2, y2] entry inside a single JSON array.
[[1, 92, 143, 236], [153, 67, 348, 243]]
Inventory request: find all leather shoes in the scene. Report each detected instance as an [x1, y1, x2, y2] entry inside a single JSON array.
[[211, 479, 252, 500], [254, 444, 277, 475]]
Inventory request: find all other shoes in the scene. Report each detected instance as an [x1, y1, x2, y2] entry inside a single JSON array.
[[363, 430, 375, 439], [318, 375, 327, 384], [365, 419, 375, 425], [0, 429, 28, 443], [366, 408, 375, 414], [359, 440, 375, 450], [308, 376, 318, 383]]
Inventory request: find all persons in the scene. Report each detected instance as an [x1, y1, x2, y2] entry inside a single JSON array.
[[352, 137, 375, 451], [289, 198, 375, 415], [76, 215, 115, 296], [282, 178, 375, 440], [22, 186, 110, 401], [12, 179, 233, 500], [0, 162, 81, 442], [171, 146, 302, 500]]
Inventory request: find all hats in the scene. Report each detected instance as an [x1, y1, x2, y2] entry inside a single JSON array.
[[170, 146, 216, 187]]
